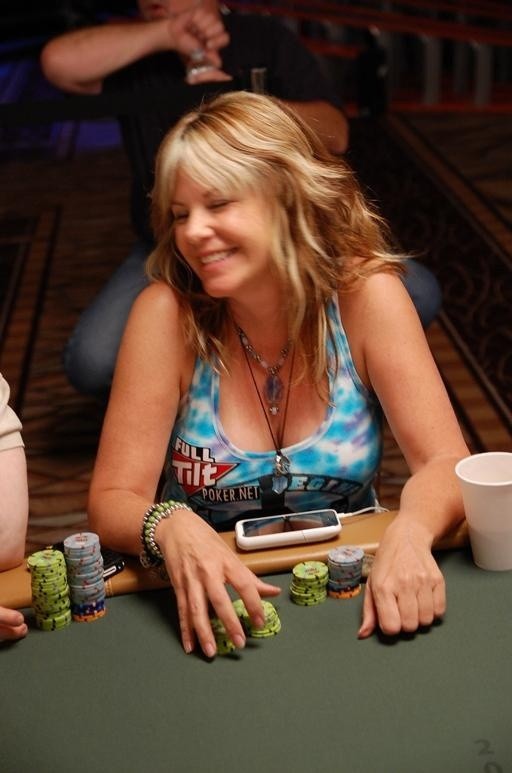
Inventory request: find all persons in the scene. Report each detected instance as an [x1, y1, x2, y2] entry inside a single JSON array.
[[37, 0, 447, 397], [85, 88, 470, 660], [1, 370, 32, 646]]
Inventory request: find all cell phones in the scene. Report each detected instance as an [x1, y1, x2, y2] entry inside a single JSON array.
[[234, 508, 342, 552]]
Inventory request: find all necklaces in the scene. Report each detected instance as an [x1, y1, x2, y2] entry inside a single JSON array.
[[225, 307, 307, 418], [233, 330, 300, 494]]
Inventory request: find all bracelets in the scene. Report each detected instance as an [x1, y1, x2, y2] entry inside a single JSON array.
[[139, 498, 194, 571]]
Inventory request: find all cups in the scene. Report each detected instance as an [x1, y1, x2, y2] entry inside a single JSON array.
[[455, 451, 512, 573]]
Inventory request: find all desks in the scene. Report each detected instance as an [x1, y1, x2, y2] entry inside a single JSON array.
[[1, 541, 512, 773]]
